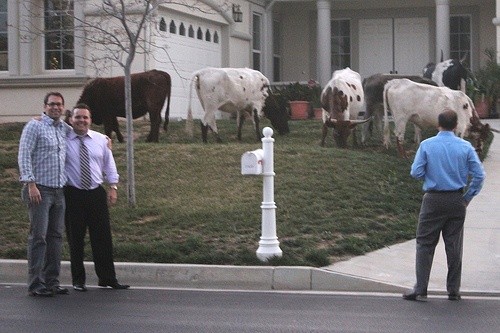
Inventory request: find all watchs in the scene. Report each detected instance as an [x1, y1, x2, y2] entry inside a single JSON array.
[[110, 186, 117, 190]]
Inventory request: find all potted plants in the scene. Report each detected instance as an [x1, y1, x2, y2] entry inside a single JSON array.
[[273, 82, 326, 120], [463, 49, 500, 119]]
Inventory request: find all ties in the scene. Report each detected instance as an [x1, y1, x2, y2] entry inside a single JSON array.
[[76, 136, 92, 190]]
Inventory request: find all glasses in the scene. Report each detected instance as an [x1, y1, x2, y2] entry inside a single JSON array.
[[45, 103, 64, 108]]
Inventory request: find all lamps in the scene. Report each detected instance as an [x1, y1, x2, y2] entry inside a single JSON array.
[[233, 3, 243, 22]]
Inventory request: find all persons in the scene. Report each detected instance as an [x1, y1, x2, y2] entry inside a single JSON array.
[[33, 103, 129, 291], [403, 109, 486, 300], [18, 93, 112, 296]]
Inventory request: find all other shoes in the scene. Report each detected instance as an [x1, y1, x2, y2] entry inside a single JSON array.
[[448, 292, 462, 300], [403, 291, 426, 299]]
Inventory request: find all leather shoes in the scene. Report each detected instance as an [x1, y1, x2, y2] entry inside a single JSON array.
[[97, 279, 130, 290], [28, 288, 53, 297], [50, 287, 69, 294], [73, 283, 85, 291]]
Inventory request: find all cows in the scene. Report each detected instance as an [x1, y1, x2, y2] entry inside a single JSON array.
[[185, 67, 289, 143], [362, 74, 437, 135], [64, 69, 172, 142], [423, 59, 467, 93], [321, 67, 375, 148], [383, 78, 486, 156]]
